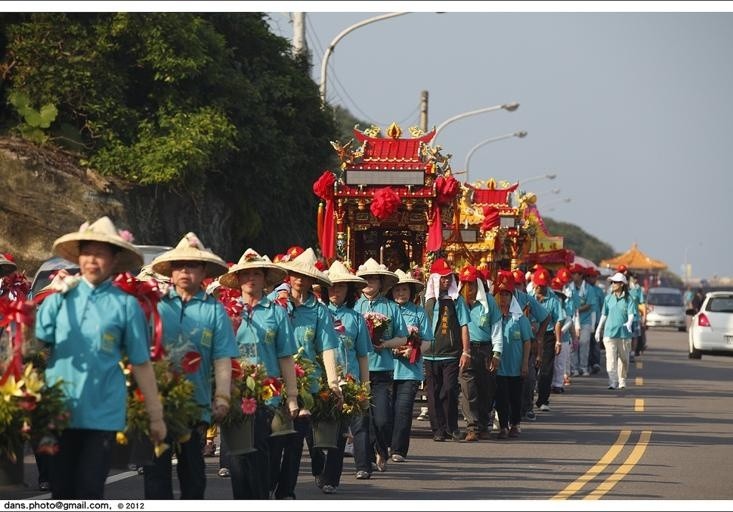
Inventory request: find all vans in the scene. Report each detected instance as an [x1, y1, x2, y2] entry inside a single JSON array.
[[28, 244, 171, 299], [645, 286, 687, 333]]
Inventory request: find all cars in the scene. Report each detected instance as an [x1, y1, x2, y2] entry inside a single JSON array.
[[686, 290, 731, 362]]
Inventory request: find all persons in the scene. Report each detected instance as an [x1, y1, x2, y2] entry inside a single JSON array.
[[691, 288, 706, 315], [683, 286, 695, 333], [0, 217, 647, 499]]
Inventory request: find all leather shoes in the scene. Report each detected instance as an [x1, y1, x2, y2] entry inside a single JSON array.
[[417, 404, 550, 441], [316, 447, 405, 492]]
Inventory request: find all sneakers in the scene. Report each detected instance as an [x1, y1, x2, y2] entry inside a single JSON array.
[[553, 363, 625, 393]]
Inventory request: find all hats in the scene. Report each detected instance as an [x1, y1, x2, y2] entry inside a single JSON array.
[[608, 273, 627, 284], [430, 259, 600, 293]]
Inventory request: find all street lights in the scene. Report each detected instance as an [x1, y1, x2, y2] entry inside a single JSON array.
[[426, 99, 572, 219]]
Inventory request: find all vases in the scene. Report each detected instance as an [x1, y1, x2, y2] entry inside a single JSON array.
[[0, 440, 24, 484]]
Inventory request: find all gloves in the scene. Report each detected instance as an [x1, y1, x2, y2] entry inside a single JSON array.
[[623, 314, 634, 333], [594, 315, 606, 342]]
